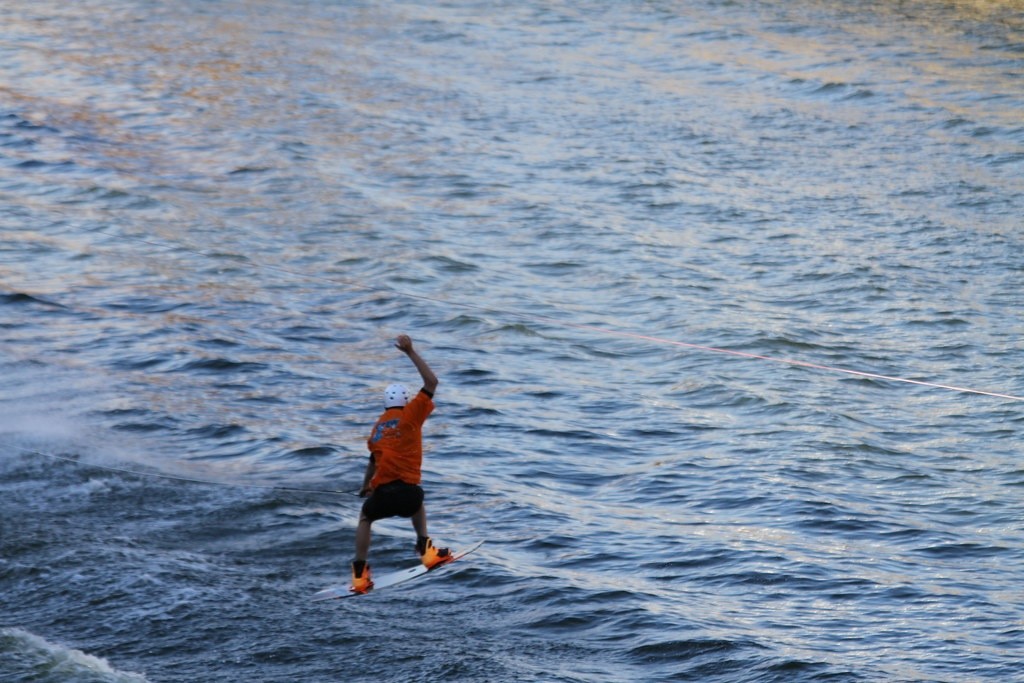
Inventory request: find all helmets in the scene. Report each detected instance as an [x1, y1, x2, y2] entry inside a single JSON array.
[[384, 384, 410, 409]]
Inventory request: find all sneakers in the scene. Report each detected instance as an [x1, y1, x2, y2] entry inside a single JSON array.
[[416, 536, 452, 568], [351, 560, 373, 594]]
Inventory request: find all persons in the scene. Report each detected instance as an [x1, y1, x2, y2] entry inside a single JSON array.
[[351, 335, 451, 593]]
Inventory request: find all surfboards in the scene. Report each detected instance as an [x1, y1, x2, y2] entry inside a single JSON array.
[[310, 539, 484, 603]]
